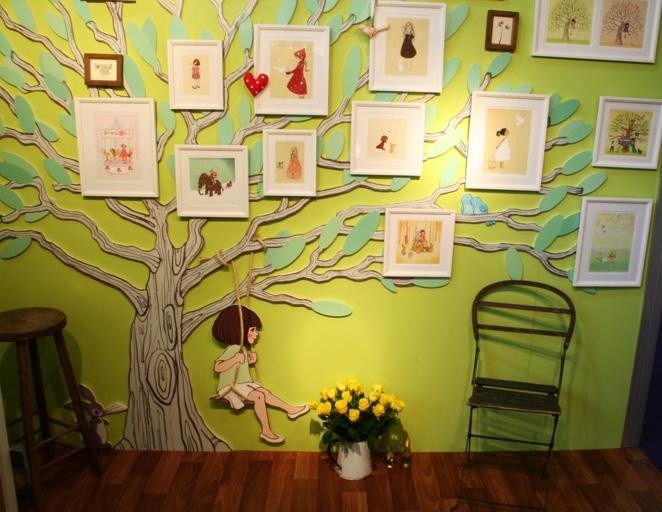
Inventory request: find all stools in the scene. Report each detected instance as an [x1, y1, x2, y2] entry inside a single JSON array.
[[0, 305, 103, 510]]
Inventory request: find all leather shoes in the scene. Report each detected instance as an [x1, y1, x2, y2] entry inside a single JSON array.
[[287, 404, 311, 420], [260, 432, 286, 446]]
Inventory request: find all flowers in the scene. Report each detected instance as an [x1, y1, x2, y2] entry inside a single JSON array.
[[301, 373, 409, 470]]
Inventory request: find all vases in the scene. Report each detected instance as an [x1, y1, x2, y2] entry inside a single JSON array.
[[332, 435, 378, 483]]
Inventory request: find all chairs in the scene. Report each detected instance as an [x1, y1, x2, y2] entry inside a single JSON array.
[[452, 279, 580, 511]]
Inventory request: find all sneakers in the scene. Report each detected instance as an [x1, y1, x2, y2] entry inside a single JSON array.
[[192, 84, 200, 90]]
